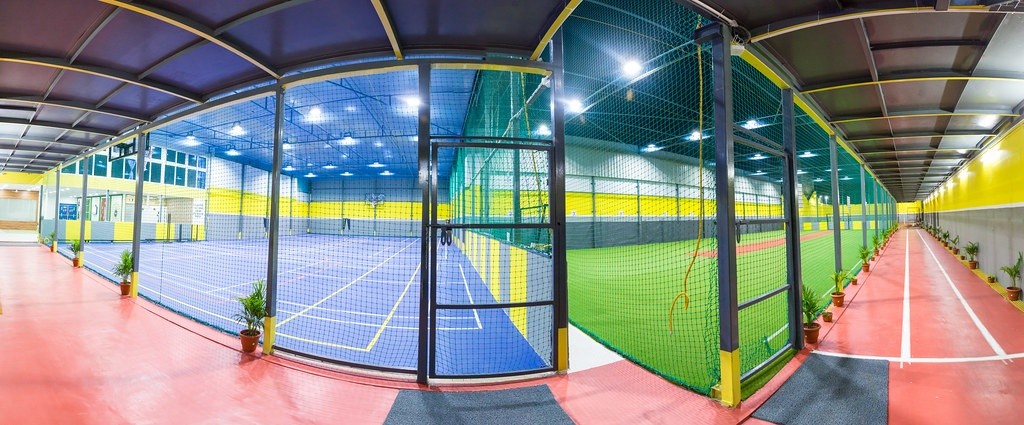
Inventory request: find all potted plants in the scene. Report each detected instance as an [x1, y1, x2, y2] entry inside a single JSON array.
[[822, 302, 833, 322], [50, 231, 56, 251], [802, 283, 821, 345], [829, 267, 850, 307], [925, 221, 965, 260], [871, 225, 900, 261], [231, 279, 270, 352], [68, 240, 84, 268], [1002, 252, 1024, 301], [845, 272, 857, 286], [859, 245, 871, 272], [964, 239, 981, 271], [111, 247, 133, 296], [987, 271, 996, 283]]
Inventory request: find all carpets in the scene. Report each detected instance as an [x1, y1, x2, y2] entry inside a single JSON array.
[[383, 383, 577, 424], [752, 349, 890, 424]]
